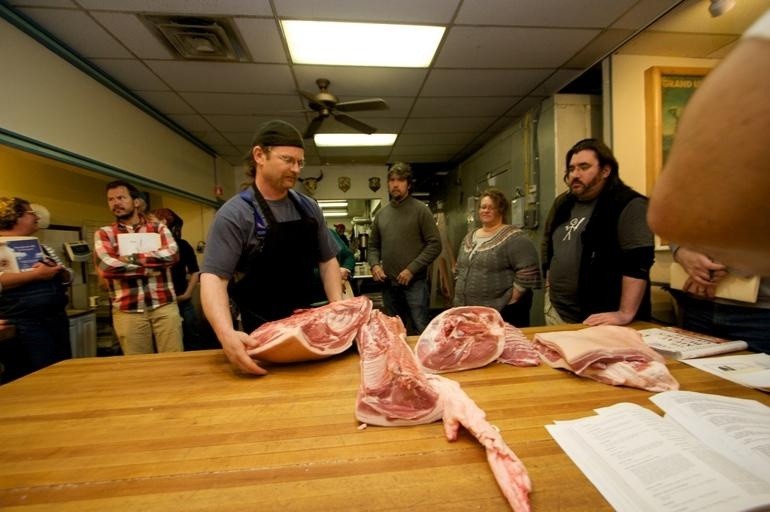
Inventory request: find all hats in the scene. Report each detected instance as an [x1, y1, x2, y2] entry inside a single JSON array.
[[254, 120, 304, 147], [334, 224, 345, 231]]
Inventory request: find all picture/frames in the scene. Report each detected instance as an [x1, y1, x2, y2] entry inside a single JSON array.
[[645, 65, 719, 199]]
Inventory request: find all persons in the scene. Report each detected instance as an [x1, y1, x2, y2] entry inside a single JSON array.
[[452, 187, 543, 327], [147, 205, 216, 349], [0, 195, 77, 384], [539, 138, 660, 328], [663, 237, 770, 312], [314, 225, 357, 305], [333, 223, 349, 245], [642, 1, 770, 279], [195, 116, 345, 378], [365, 159, 444, 339], [92, 178, 189, 356]]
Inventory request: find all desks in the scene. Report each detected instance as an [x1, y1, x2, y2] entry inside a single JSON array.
[[0, 319, 770, 512]]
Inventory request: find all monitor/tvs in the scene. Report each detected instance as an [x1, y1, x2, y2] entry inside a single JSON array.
[[388, 161, 451, 199]]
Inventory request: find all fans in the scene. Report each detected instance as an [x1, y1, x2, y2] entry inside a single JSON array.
[[252, 78, 391, 138]]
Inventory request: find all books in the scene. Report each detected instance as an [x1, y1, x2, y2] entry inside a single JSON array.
[[117, 233, 162, 276], [0, 236, 46, 273], [726, 366, 770, 392], [638, 326, 750, 359], [677, 352, 769, 390], [544, 390, 770, 512], [669, 263, 761, 304]]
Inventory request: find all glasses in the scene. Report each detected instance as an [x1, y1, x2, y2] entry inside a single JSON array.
[[267, 148, 305, 169]]
